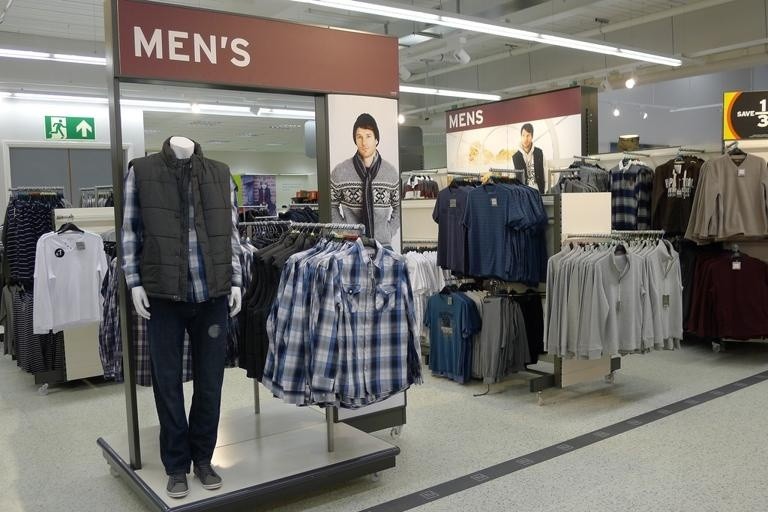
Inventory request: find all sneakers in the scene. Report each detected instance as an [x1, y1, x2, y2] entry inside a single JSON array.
[[167, 472, 191, 498], [194, 465, 222, 490]]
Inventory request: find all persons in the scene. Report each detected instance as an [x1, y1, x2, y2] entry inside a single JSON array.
[[329, 112, 400, 252], [505, 123, 549, 194], [122, 135, 241, 496]]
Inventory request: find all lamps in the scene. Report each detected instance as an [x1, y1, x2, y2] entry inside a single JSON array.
[[399, 59, 502, 103], [450, 36, 472, 65], [623, 75, 638, 92], [295, 0, 682, 69], [398, 65, 410, 84], [610, 102, 649, 123]]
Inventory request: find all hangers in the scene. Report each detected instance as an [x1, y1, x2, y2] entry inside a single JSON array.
[[237, 204, 378, 253], [727, 240, 742, 261], [55, 212, 87, 242], [447, 169, 526, 192], [405, 167, 434, 183], [8, 186, 114, 204], [3, 274, 34, 299], [561, 230, 669, 257], [558, 142, 746, 186], [398, 236, 512, 301]]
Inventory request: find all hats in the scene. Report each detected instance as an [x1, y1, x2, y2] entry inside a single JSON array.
[[353, 113, 380, 146]]
